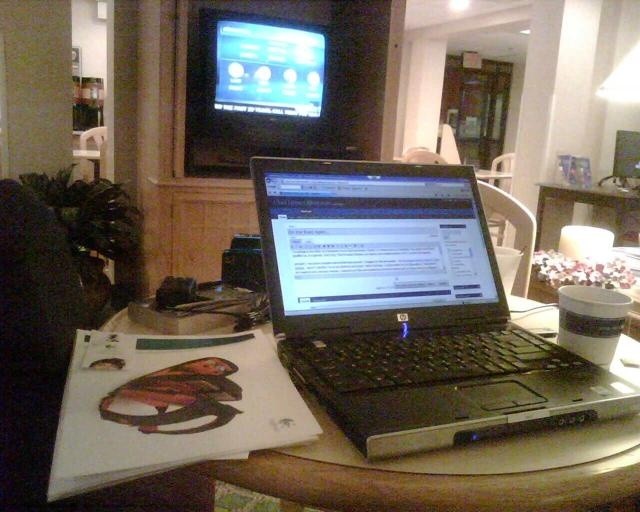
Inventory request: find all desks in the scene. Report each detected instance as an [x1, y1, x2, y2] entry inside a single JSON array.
[[73, 149, 101, 162], [526, 247, 640, 344], [534, 181, 640, 251], [94, 282, 640, 512]]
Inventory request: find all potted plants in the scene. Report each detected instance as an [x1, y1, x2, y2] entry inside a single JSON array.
[[15, 161, 143, 331]]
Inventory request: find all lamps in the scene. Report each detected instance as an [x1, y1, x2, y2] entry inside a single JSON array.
[[594, 37, 640, 106]]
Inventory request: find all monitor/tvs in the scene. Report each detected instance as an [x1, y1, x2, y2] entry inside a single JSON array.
[[196, 7, 340, 152], [613, 129, 640, 190]]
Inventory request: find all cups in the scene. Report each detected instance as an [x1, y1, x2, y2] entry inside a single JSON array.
[[551, 284, 635, 366], [493, 245, 525, 304]]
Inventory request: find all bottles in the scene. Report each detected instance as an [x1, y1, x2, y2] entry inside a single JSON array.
[[73, 84, 105, 131]]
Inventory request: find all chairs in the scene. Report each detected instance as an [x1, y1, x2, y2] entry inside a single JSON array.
[[400, 145, 538, 298], [0, 177, 217, 512], [79, 126, 108, 183]]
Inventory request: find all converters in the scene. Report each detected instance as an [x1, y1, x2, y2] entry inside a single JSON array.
[[156, 276, 196, 305]]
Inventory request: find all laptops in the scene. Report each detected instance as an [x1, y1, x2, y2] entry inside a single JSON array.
[[248, 156, 640, 462]]
